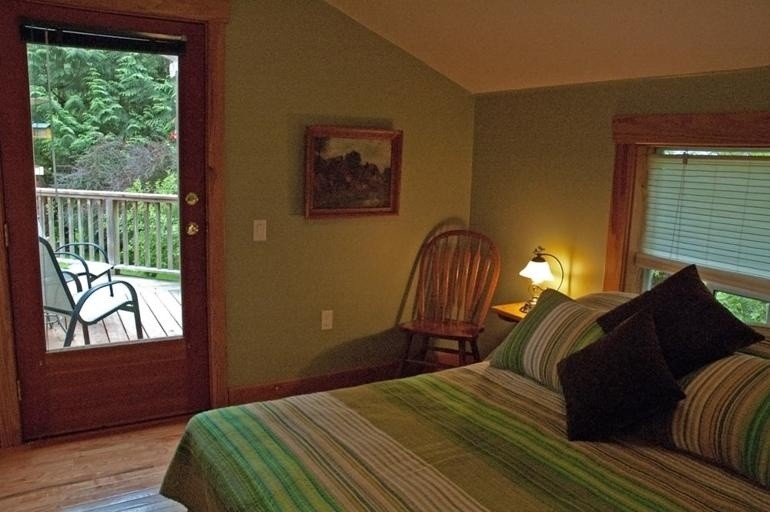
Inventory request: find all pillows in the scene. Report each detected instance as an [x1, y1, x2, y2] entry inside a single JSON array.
[[570, 290, 638, 313], [594, 263, 766, 379], [489, 287, 611, 393], [555, 305, 688, 443], [742, 321, 769, 361], [627, 352, 769, 493]]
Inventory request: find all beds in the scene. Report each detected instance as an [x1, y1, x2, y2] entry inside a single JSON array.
[[160, 326, 770, 512]]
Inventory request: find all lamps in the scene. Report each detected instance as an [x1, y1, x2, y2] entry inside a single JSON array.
[[518, 245, 565, 312]]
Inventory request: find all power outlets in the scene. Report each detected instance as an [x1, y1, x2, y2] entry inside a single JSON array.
[[319, 308, 334, 332]]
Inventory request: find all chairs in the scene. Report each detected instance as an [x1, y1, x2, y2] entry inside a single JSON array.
[[39, 236, 143, 347], [389, 231, 501, 379], [53, 242, 114, 297]]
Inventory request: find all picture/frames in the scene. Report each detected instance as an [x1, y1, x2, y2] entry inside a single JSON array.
[[304, 123, 403, 221]]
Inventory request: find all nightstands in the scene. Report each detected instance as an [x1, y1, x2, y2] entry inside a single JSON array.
[[492, 301, 528, 324]]
